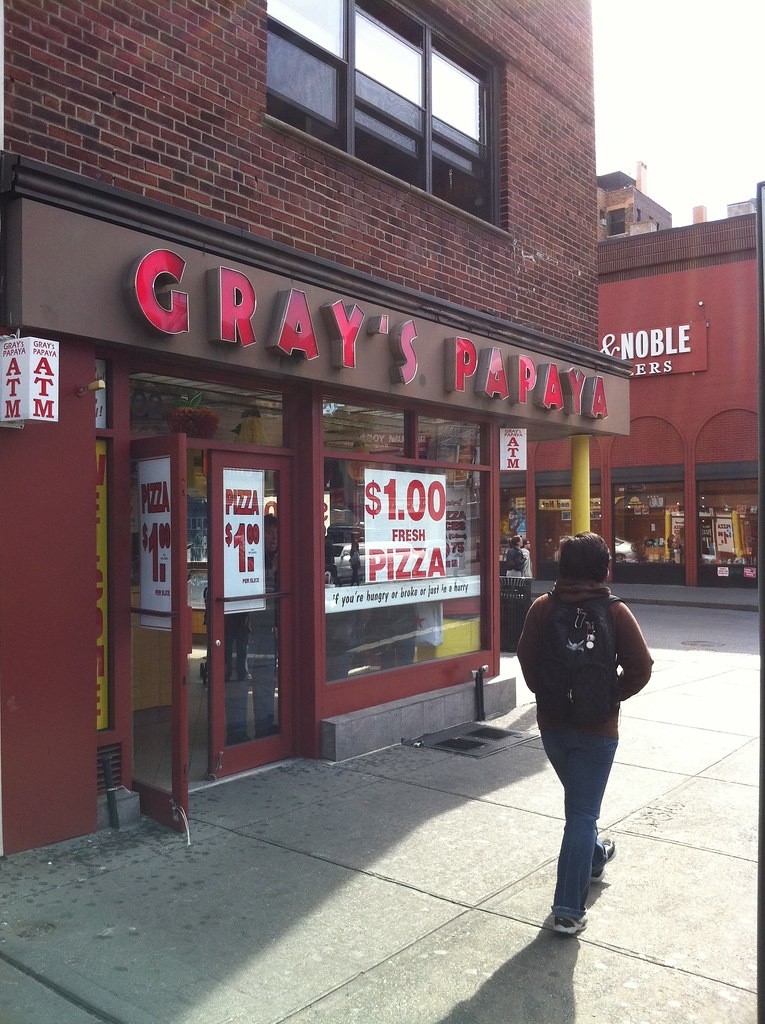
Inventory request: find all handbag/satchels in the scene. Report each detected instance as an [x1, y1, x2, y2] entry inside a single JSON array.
[[512, 549, 525, 571]]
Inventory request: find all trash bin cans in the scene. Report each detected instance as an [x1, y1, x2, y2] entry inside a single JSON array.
[[500, 576, 533, 653]]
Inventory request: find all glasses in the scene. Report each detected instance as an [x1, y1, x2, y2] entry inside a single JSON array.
[[526, 542, 531, 545]]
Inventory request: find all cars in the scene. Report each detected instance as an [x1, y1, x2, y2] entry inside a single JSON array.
[[615, 537, 637, 563], [332, 543, 365, 578]]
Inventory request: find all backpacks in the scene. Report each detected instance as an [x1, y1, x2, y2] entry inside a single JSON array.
[[536, 590, 624, 723]]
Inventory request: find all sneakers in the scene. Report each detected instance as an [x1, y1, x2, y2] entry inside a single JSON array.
[[553, 914, 588, 934], [591, 838, 617, 877]]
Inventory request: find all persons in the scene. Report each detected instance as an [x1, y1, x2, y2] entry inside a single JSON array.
[[225, 613, 252, 681], [516, 532, 654, 934], [225, 597, 276, 746], [266, 514, 279, 582], [667, 527, 684, 562], [349, 539, 362, 584], [191, 527, 204, 561], [506, 535, 532, 577]]
